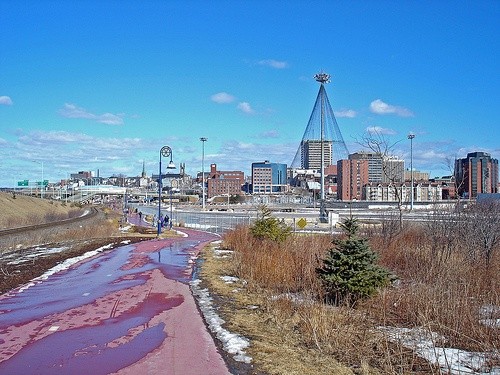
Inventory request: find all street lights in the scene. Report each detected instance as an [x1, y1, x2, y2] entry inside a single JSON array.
[[408, 132, 415, 211], [65, 177, 99, 204], [200, 135, 208, 211], [158, 145, 177, 237], [32, 160, 44, 199], [314, 70, 332, 225]]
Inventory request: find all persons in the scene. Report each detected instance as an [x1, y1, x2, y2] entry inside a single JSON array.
[[139, 212, 142, 218], [160, 216, 164, 226], [136, 208, 137, 213], [164, 214, 169, 226]]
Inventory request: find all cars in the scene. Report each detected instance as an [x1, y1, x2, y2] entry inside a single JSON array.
[[82, 192, 87, 196], [53, 196, 61, 199]]
